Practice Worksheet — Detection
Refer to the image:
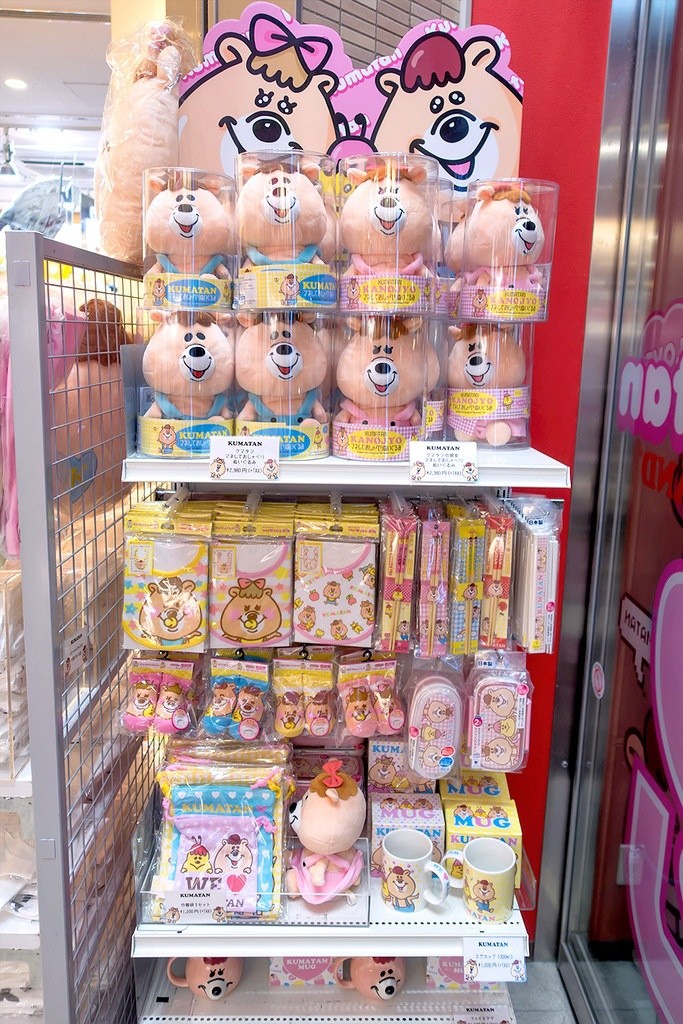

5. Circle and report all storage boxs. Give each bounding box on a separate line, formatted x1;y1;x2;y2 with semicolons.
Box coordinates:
371;792;445;879
367;736;435;797
439;768;510;806
443;799;522;889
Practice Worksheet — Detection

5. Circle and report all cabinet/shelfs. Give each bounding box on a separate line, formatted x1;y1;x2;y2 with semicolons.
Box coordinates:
1;230;168;1024
121;445;570;1024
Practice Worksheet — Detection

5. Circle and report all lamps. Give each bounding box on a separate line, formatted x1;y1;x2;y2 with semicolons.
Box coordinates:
0;127;15;174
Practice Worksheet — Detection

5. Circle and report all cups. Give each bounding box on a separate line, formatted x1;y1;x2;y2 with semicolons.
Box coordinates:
166;958;244;1002
441;837;517;924
382;828;450;911
332;957;406;1001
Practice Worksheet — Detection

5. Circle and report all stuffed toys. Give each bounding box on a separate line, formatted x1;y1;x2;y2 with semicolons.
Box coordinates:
44;299;143;519
231;308;328;428
335;312;425;427
94;43;180;263
467;185;547;289
340;161;426;275
283;757;367;908
144;170;236;278
142;308;232;420
238;159;328;268
445;320;526;448
